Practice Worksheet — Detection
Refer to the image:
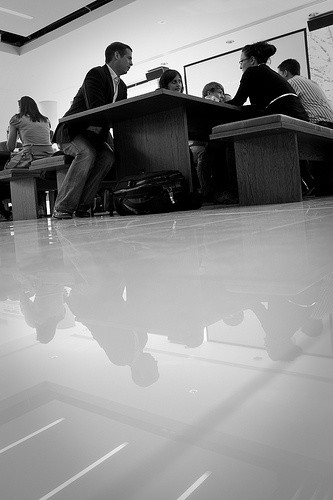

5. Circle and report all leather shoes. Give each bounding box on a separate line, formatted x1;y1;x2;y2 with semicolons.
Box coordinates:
52;207;72;219
75;209;90;217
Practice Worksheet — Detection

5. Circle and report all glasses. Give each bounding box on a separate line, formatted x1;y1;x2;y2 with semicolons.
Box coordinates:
239;57;251;64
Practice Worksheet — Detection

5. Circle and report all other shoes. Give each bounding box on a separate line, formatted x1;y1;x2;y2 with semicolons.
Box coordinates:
38;208;45;217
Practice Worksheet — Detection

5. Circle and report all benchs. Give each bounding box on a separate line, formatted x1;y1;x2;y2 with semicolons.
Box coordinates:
29;155;94;217
0;169;42;221
209;114;333;207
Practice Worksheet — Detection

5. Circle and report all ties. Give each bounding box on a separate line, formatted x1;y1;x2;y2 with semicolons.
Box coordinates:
112;77;119;103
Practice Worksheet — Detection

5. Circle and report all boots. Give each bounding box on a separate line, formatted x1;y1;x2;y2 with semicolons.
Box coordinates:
301;177;315;196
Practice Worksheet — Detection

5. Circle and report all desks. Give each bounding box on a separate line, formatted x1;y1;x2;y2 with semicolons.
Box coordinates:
59;88;263;191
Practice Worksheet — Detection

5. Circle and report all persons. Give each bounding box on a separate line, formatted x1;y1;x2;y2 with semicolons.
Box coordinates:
225;42;317;196
4;96;53;217
277;59;333;129
156;69;202;194
189;82;237;204
51;42;133;218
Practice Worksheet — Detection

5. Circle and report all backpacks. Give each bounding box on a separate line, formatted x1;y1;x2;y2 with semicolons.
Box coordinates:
113;170;202;216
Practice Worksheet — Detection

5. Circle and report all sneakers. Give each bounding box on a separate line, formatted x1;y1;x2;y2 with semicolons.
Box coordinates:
215;190;238;204
